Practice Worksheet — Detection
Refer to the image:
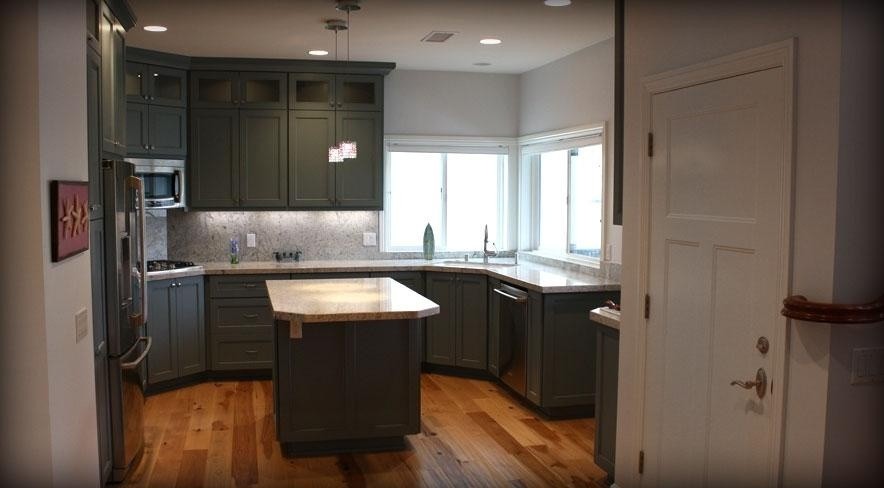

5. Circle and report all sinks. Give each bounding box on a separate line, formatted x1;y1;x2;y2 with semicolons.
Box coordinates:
433;261;521;267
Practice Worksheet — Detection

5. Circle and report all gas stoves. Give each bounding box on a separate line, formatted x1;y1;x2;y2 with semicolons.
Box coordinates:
135;259;199;273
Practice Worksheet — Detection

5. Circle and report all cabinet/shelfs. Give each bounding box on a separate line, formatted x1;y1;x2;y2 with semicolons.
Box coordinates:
87;0;116;487
127;64;187;155
206;275;291;373
142;276;205;393
424;272;488;370
289;72;382;208
98;0;137;160
191;72;287;208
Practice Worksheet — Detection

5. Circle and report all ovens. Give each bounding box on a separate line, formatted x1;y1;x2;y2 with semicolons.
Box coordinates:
493;281;528;399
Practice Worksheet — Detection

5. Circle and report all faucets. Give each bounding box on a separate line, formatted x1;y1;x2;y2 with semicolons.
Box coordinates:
484;224;488;263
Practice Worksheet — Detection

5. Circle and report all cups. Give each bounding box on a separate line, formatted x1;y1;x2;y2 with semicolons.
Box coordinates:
230;253;239;264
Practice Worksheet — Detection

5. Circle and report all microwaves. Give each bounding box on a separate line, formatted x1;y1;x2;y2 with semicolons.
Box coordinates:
125;159;189;209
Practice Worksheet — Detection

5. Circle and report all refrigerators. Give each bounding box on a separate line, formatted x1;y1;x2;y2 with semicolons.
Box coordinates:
103;158;153;472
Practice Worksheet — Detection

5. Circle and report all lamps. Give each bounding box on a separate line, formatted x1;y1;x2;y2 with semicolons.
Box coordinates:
324;0;361;163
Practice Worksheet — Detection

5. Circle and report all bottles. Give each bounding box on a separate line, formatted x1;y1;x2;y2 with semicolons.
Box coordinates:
423;224;435;260
